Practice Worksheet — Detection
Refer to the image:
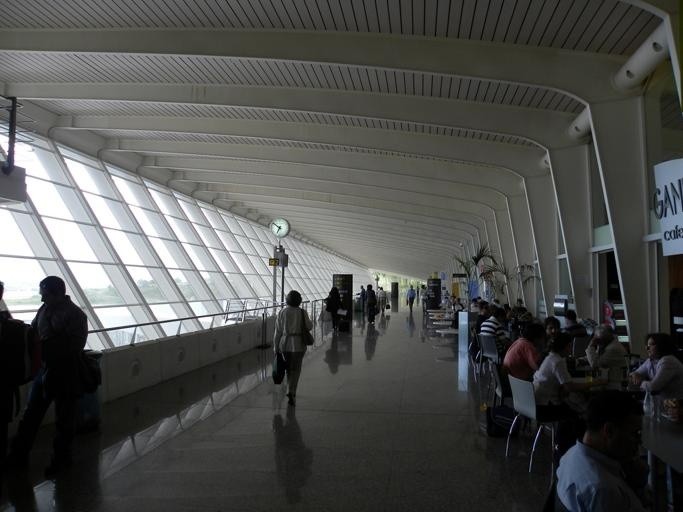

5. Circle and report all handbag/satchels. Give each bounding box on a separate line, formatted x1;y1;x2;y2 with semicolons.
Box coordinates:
386;303;390;309
272;355;285;384
80;357;99;397
302;327;314;346
336;309;348;316
486;405;518;438
367;296;376;304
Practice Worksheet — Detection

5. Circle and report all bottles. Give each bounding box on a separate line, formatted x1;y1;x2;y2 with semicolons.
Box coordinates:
642;387;655;417
619;367;629;388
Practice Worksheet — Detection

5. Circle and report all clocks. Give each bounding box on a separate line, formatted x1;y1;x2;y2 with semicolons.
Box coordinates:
270;217;290;239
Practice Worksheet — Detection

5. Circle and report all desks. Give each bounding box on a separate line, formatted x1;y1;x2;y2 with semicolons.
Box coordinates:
639;415;683;491
427;309;458;337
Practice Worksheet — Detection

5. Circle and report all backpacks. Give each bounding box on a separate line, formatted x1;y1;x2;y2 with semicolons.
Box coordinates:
2;313;43;386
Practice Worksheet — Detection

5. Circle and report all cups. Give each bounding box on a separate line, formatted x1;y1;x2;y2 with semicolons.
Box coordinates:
584;366;593;384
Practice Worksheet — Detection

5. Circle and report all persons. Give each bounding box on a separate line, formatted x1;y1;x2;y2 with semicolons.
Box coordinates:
356;284;389;324
326;287;343;332
0;282;20;512
439;295;683;512
273;290;313;405
419;285;428;312
407;284;416;312
8;276;88;478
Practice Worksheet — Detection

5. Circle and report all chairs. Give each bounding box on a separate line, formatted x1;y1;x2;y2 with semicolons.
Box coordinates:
470;324;593;485
